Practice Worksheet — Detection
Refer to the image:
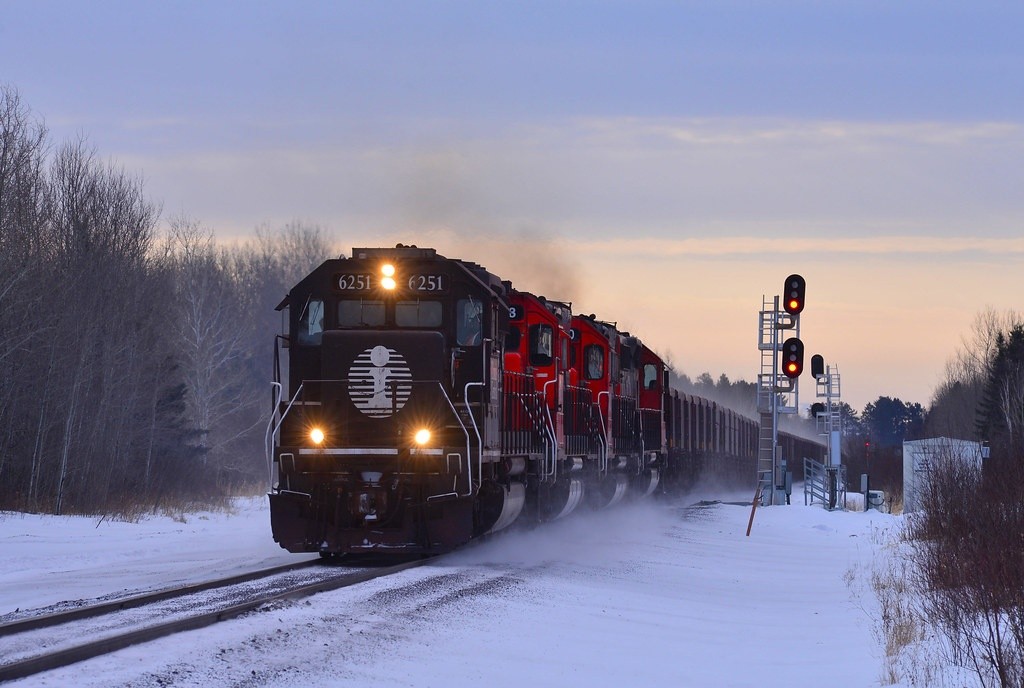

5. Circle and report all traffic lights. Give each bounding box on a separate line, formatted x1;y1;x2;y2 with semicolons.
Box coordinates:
784;275;804;315
864;440;872;458
782;336;803;378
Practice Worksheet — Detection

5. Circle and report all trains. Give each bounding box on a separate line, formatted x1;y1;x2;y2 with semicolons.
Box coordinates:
269;245;826;566
